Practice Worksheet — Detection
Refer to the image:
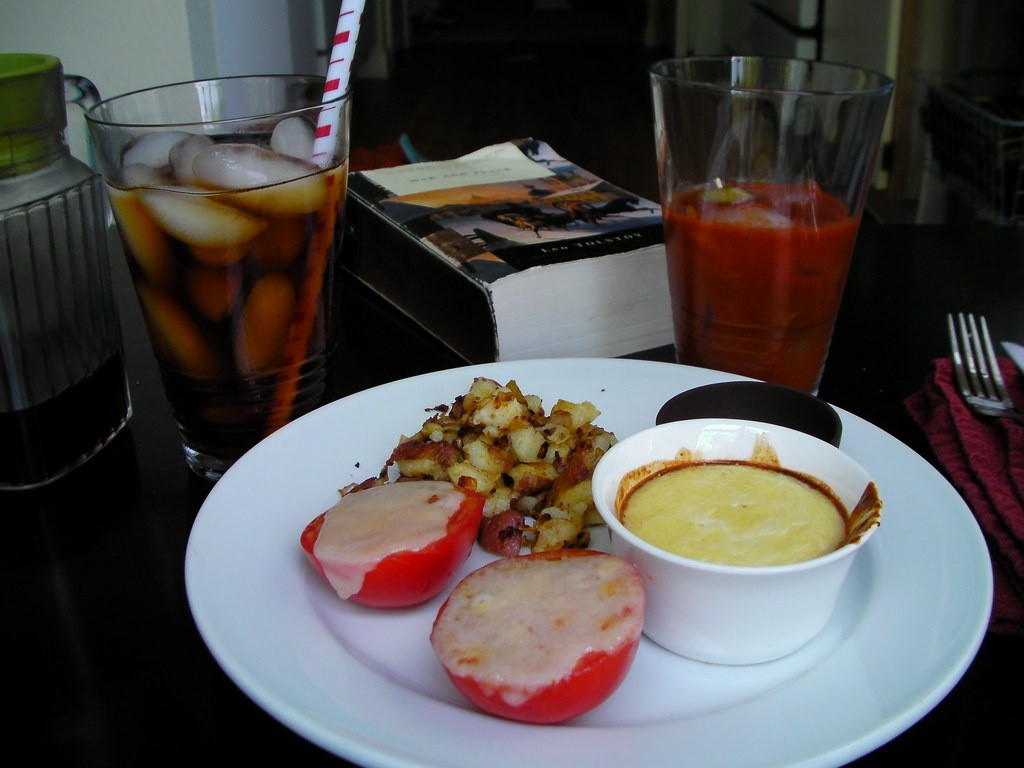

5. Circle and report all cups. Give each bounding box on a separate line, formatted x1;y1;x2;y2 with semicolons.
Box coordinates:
86;73;355;483
648;54;897;400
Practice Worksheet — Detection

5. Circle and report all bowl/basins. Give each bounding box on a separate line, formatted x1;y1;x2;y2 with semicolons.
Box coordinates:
588;418;882;666
656;381;843;454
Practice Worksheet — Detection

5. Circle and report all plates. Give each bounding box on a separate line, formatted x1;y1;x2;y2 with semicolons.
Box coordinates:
182;355;994;768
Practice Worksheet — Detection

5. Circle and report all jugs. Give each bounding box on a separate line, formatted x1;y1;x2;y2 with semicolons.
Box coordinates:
0;53;133;491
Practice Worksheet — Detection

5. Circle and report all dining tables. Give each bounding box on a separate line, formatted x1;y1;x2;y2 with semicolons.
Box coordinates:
0;224;1024;768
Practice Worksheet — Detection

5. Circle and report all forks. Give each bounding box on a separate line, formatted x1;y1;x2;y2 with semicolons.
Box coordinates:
947;311;1024;504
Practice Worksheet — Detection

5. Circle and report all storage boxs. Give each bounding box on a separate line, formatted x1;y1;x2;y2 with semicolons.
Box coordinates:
907;70;1023;227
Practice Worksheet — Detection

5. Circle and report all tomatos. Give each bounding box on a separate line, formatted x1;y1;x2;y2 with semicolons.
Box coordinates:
429;548;648;723
299;479;485;608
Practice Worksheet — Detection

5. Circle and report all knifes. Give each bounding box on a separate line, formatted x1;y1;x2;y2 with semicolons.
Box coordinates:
999;340;1024;374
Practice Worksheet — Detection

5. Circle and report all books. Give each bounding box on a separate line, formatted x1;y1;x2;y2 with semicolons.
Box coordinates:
339;136;688;370
